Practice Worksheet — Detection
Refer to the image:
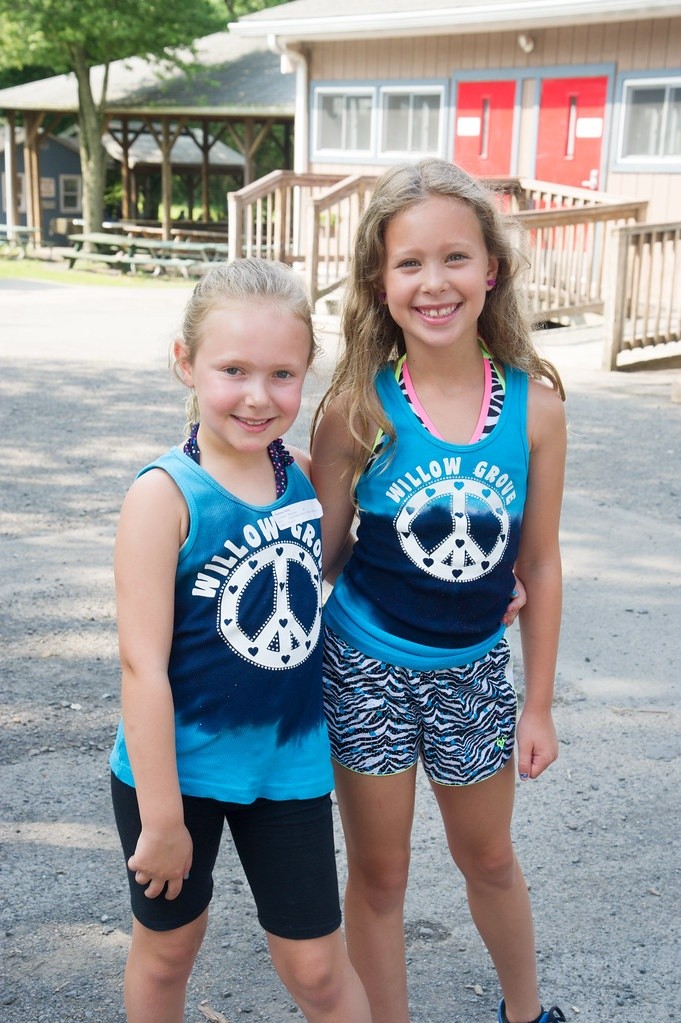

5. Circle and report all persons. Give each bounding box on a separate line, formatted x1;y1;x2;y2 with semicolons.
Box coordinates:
311;157;573;1023
104;257;527;1023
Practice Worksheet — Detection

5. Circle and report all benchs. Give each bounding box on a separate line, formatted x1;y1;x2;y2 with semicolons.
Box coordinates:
0;216;293;279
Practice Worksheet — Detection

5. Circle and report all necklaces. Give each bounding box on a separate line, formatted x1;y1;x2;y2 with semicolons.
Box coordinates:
184;422;294;502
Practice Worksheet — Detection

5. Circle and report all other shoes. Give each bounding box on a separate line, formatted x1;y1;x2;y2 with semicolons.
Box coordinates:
496;997;567;1023
